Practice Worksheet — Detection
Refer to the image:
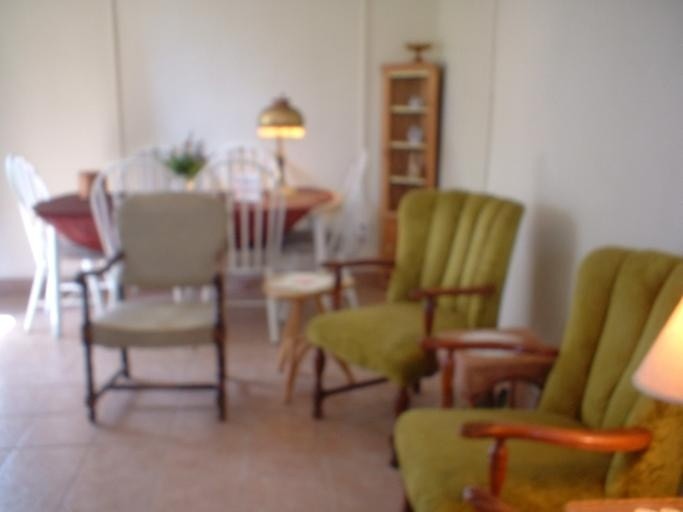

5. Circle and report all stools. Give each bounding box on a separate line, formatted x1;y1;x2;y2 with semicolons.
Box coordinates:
261;271;355;402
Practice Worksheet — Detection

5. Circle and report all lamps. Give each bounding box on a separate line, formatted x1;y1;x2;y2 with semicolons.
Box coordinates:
254;96;305;200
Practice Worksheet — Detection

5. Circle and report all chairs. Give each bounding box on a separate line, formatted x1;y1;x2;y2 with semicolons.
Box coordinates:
304;189;523;470
74;192;230;422
388;243;683;511
4;138;366;343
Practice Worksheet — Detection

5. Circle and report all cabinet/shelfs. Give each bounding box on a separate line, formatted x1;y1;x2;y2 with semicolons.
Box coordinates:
379;62;443;276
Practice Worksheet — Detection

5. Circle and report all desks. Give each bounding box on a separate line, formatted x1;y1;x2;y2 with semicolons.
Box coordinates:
434;321;559;415
